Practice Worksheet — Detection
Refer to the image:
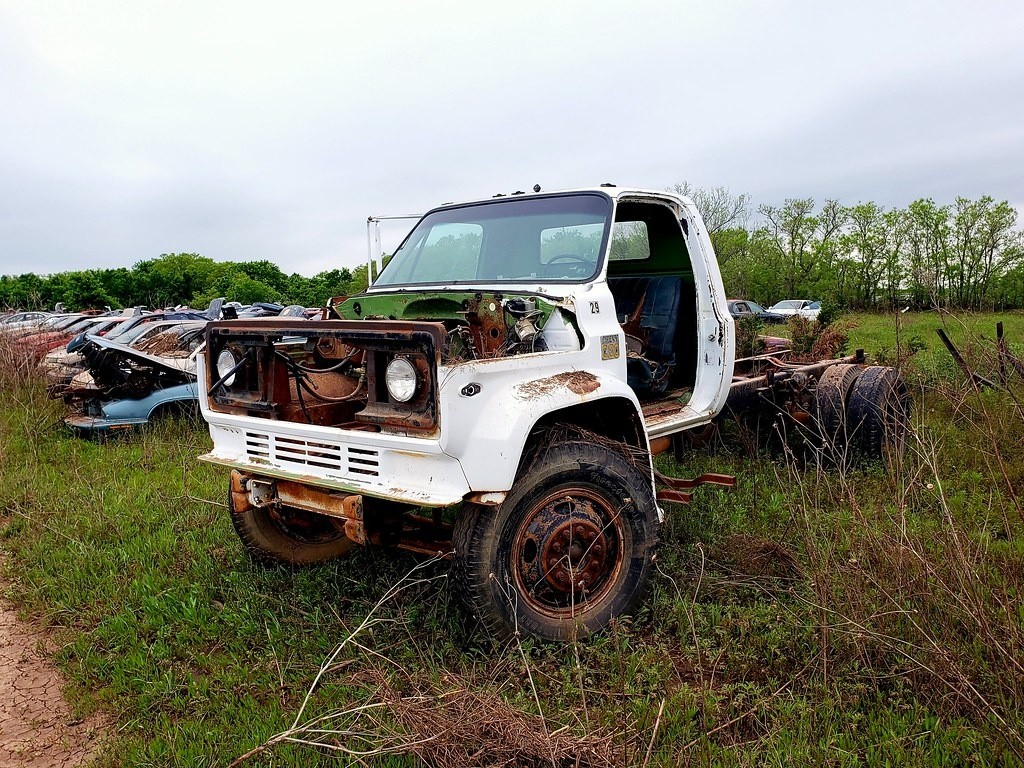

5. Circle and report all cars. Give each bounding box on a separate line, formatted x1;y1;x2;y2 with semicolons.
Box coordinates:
726;299;790;326
764;299;823;322
0;296;337;446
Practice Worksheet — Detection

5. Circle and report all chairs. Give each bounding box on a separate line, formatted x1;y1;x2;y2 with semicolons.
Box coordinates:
618;274;683;400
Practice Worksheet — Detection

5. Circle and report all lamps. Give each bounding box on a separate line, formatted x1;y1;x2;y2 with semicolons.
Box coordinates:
383;356;424;404
216;348;246;388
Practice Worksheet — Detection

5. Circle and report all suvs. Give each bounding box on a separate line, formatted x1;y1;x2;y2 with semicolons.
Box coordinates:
186;183;910;661
